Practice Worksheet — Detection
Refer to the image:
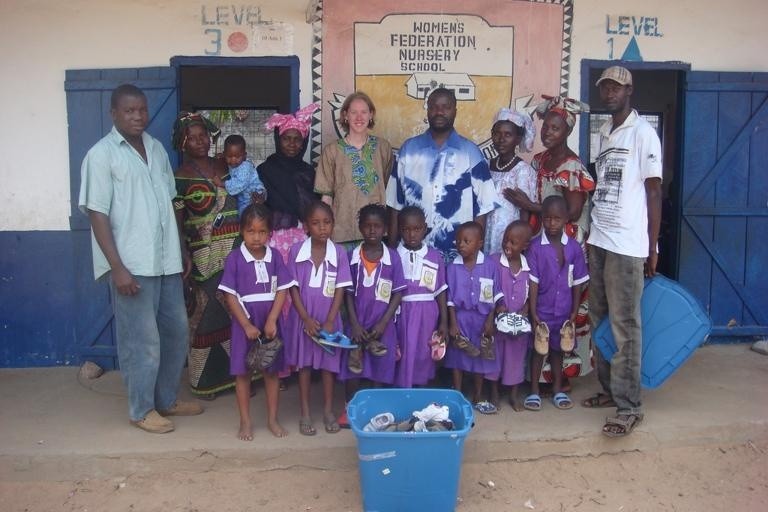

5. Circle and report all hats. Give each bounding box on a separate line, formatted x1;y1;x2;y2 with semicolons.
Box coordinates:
595;66;633;89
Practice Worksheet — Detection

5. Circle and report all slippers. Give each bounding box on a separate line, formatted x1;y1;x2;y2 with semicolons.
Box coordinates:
299;420;316;435
324;419;340;433
523;394;542;411
549;393;573;409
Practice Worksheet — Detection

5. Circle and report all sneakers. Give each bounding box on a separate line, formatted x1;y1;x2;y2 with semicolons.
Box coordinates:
495;312;532;336
413;403;448;423
480;333;496;360
129;408;174;434
363;413;395;432
156;399;204;416
451;334;480;358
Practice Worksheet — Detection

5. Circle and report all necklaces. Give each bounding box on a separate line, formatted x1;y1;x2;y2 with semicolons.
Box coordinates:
346;136;367;151
496;154;517;171
189;160;212;178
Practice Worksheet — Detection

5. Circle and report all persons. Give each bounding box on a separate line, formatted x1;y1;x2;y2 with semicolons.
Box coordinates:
337;204;408;428
482;108;537;251
173;112;269;401
218;203;292;441
582;66;663;437
76;84;205;435
313;91;395;252
524;195;590;410
501;96;596;399
446;221;504;417
214;134;268;218
491;220;530;413
395;206;450;388
256;102;317;392
384;89;501;264
286;199;354;435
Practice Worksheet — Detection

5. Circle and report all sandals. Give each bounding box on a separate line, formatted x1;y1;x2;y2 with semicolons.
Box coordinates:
428;330;447;362
582;393;617;408
302;328;358;356
364;336;389;357
246;334;283;372
347;341;364;375
395;344;402;362
602;413;644;438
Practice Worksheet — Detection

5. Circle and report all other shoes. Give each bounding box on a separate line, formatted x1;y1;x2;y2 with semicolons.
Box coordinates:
387;418;454;433
473;401;497;414
337;413;351;429
559;320;576;352
534;322;550;355
